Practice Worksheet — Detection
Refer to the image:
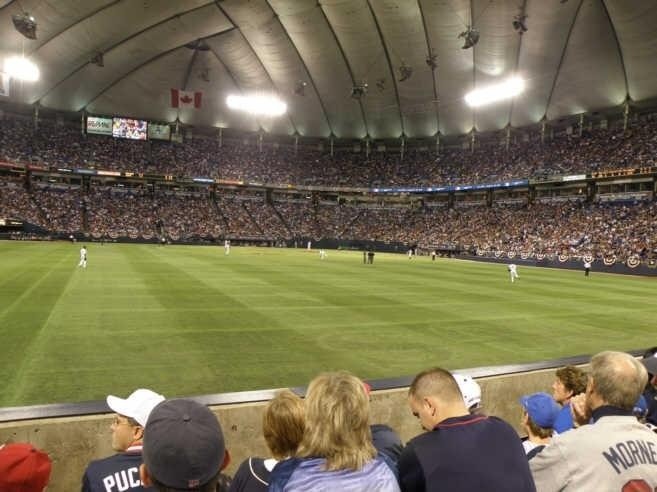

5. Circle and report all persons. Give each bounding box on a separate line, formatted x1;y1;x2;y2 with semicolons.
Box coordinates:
1;112;657;264
78;245;87;268
507;264;520;285
584;261;592;278
0;349;657;492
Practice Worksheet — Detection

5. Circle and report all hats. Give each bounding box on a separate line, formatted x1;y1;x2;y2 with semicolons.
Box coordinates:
520;392;560;427
0;443;52;492
106;389;166;429
453;374;481;410
141;400;226;486
632;392;647;413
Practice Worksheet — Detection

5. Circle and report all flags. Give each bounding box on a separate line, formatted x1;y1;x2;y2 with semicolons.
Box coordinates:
169;87;203;109
